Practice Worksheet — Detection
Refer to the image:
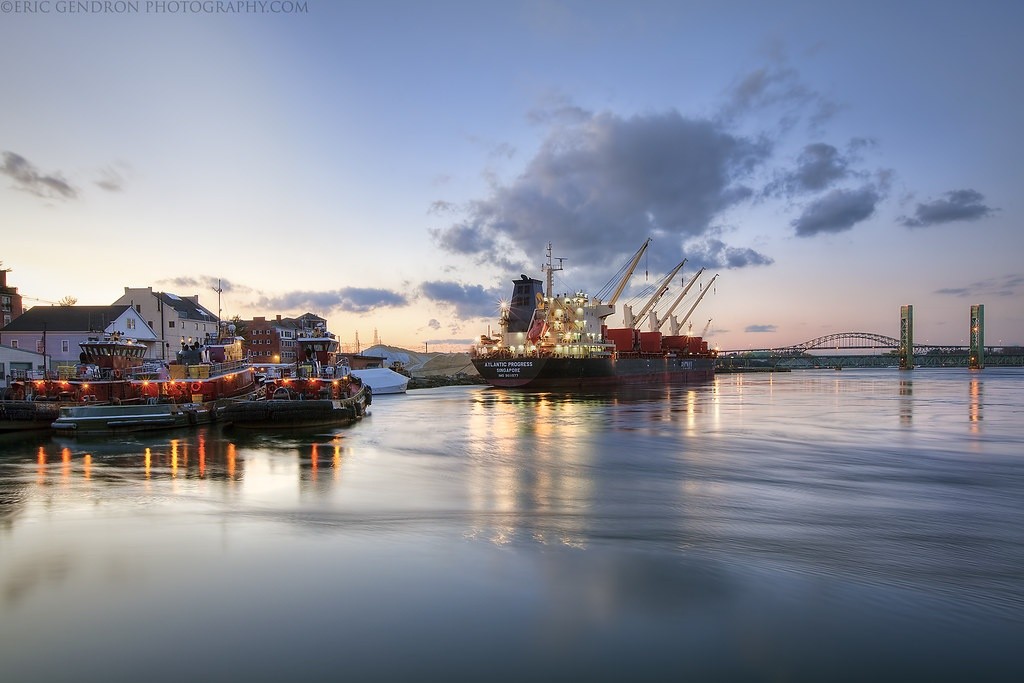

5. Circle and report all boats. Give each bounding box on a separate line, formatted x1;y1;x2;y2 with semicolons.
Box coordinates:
50;276;267;434
466;241;718;390
212;371;373;430
0;320;169;432
527;319;550;346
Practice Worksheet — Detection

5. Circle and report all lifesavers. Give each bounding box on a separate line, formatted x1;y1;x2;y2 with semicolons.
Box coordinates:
190;382;202;393
115;369;120;378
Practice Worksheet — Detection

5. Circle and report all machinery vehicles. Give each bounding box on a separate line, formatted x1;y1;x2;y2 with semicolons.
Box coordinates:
620;257;688;355
582;236;655;352
645;267;719;356
